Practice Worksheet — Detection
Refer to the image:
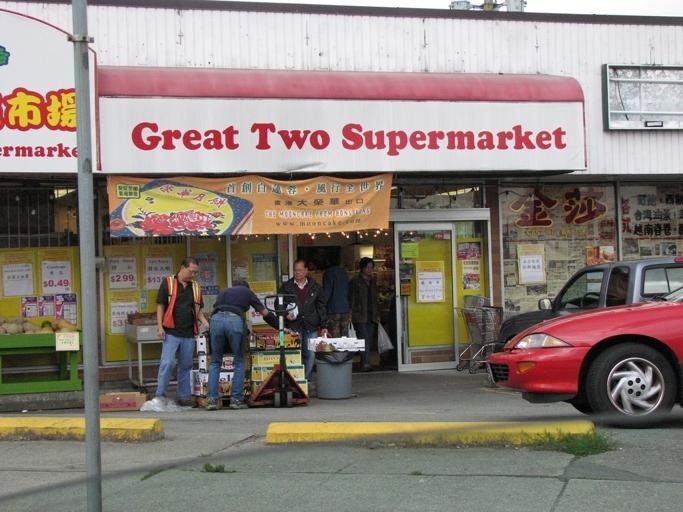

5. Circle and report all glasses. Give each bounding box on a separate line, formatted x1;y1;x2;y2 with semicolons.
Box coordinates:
186;268;199;276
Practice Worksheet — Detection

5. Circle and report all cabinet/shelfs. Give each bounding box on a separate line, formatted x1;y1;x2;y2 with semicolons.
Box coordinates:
0;329;83;394
125;324;178;388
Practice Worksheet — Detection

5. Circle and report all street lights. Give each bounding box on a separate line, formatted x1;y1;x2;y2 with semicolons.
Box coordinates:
448;0;527;14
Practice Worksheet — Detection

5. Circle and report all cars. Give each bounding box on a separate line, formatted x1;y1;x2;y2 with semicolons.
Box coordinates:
489;285;683;429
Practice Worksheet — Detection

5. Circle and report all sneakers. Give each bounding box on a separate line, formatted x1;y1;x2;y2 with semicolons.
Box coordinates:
204;396;218;410
176;399;198;408
229;398;249;409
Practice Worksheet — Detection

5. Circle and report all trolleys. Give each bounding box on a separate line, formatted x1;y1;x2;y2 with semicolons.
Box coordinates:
456;304;516;375
247;294;311;408
124;320;178;395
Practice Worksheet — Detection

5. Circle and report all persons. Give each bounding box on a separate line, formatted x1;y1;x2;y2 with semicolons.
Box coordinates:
274;259;327;383
155;257;210;408
206;280;289;410
321;254;351;338
348;257;381;371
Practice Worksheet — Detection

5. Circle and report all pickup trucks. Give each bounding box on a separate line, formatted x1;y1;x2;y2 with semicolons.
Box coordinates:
497;257;683;352
